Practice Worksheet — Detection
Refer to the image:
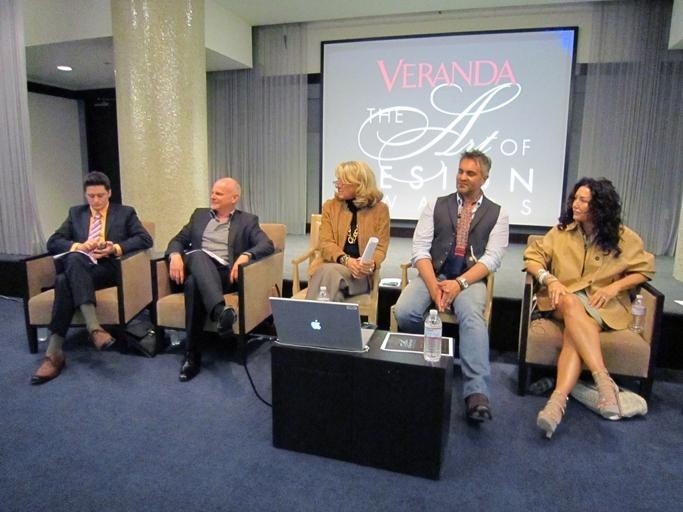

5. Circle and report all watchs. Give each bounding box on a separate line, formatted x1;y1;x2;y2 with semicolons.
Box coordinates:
456;277;469;290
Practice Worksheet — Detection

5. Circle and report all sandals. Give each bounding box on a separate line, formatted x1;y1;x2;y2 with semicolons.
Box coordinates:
592;368;622;421
536;391;570;438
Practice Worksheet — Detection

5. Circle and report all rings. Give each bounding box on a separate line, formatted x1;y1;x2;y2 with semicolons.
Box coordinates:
602;296;606;300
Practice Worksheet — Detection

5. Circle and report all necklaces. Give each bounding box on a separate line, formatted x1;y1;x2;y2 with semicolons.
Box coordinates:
347;222;358;245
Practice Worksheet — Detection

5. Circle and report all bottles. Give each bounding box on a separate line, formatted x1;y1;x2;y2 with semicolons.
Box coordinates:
629;294;647;334
423;310;443;363
316;286;330;302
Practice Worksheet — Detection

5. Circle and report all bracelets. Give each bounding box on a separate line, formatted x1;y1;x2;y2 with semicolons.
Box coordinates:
339;254;350;265
536;269;546;280
539;271;549;286
243;252;253;260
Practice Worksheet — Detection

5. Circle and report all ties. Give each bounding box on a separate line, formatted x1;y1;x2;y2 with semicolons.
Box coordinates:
87;213;102;248
456;201;474;257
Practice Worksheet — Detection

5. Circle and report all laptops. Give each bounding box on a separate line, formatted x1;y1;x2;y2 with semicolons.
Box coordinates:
268;297;374;349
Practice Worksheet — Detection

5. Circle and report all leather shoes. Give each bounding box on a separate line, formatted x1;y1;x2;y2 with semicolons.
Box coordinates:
177;356;202;383
217;308;236;341
466;395;492;422
91;328;116;352
30;355;67;383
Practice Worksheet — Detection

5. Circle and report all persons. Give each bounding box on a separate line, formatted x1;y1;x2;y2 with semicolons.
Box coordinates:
303;160;390;303
30;172;152;385
392;149;510;422
162;178;274;381
520;176;655;439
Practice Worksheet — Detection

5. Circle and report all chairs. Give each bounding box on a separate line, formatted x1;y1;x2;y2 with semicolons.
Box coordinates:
514;230;667;396
290;212;383;329
19;219;159;356
149;221;286;366
386;255;494;360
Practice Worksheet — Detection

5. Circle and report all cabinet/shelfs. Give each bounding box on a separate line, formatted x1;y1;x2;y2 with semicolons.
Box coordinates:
268;330;456;480
